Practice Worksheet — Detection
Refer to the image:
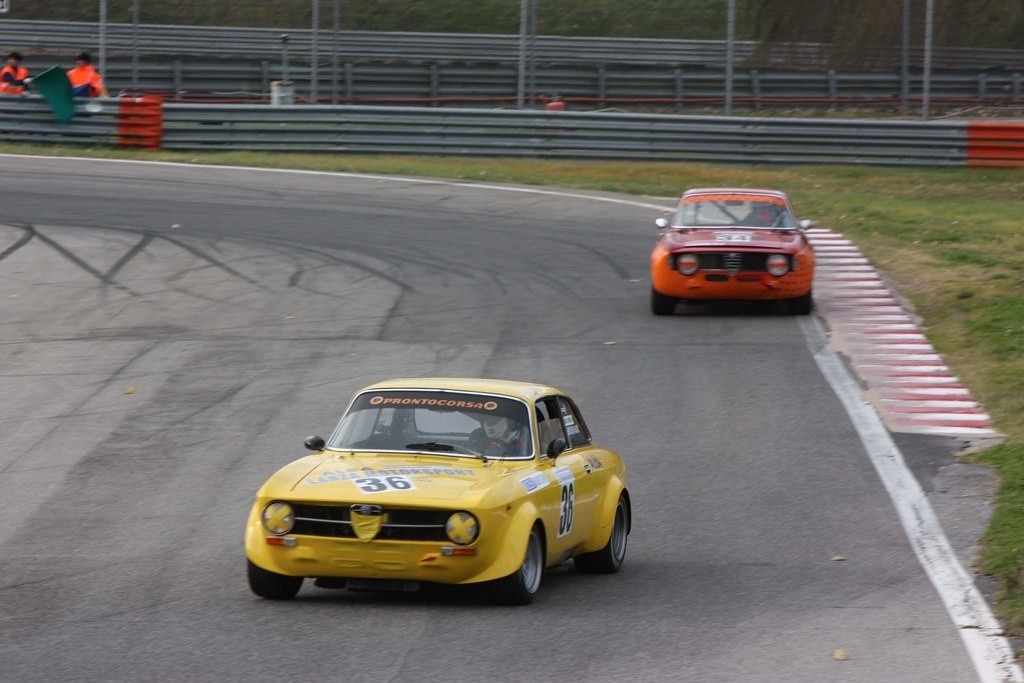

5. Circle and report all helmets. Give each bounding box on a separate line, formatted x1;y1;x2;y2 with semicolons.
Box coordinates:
752;199;779;226
483;398;520;446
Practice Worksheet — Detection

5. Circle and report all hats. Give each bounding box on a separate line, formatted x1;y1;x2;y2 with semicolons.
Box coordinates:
75;51;89;61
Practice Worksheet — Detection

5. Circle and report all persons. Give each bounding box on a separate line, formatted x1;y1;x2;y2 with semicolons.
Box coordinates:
66;53;109;97
0;51;30;96
470;415;523;455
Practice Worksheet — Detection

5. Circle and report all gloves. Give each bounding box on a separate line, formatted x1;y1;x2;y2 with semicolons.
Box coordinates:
23;91;30;95
23;77;33;84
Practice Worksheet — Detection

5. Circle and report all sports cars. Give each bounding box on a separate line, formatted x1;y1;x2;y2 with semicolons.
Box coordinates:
244;376;632;606
649;186;818;314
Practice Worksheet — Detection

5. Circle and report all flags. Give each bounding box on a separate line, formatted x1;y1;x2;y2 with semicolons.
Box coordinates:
33;66;75;123
